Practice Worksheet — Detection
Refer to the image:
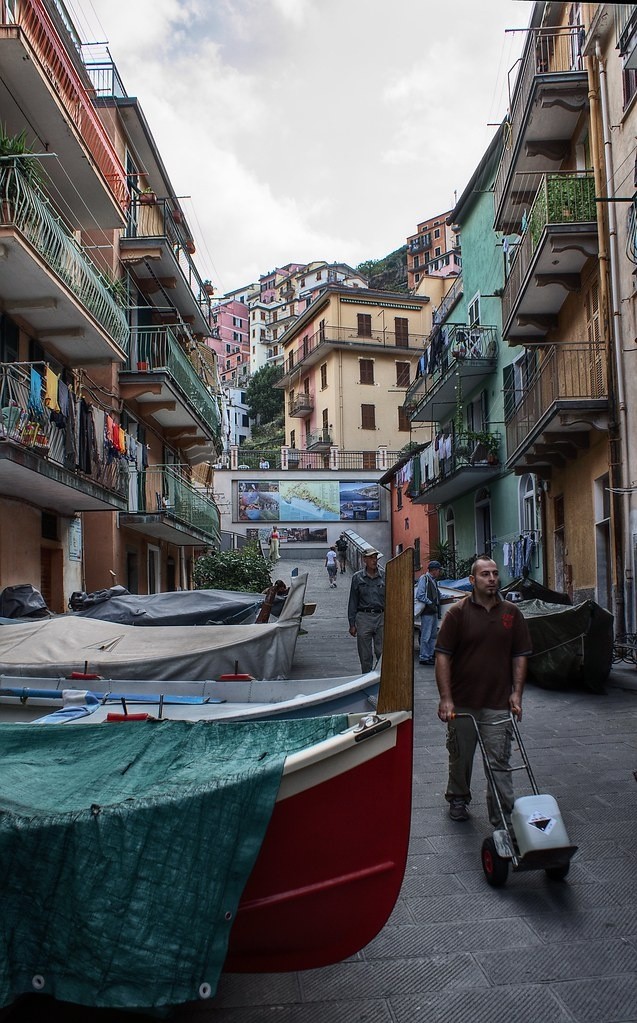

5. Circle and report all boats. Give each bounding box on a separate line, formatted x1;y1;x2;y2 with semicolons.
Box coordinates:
0;548;418;977
0;572;308;682
494;569;614;693
0;652;382;725
70;568;298;626
411;581;472;632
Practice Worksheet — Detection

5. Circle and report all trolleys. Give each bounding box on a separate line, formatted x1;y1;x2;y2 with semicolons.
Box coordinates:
451;710;579;888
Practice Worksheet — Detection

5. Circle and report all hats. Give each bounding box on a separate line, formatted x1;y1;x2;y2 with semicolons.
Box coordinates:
427;561;446;571
362;548;380;556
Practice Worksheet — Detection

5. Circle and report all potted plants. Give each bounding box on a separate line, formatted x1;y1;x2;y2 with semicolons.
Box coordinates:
487;446;499;463
136;352;149;369
139;187;157;203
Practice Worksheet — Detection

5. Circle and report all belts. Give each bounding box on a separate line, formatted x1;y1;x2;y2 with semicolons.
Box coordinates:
359;608;384;614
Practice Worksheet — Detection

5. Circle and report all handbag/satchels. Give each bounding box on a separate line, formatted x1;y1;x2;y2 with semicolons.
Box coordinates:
414;574;429;619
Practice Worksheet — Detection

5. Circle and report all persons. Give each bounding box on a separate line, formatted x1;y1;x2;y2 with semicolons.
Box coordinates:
268;525;284;566
334;534;349;574
416;561;455;664
347;548;386;674
259;457;270;469
434;555;533;844
325;547;338;588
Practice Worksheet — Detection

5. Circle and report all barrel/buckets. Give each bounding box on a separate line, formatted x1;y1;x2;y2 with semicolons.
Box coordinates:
137;362;148;370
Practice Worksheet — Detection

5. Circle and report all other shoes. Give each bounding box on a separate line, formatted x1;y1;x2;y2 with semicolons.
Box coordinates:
420;659;435;665
448;800;469;821
330;585;333;588
333;581;337;587
